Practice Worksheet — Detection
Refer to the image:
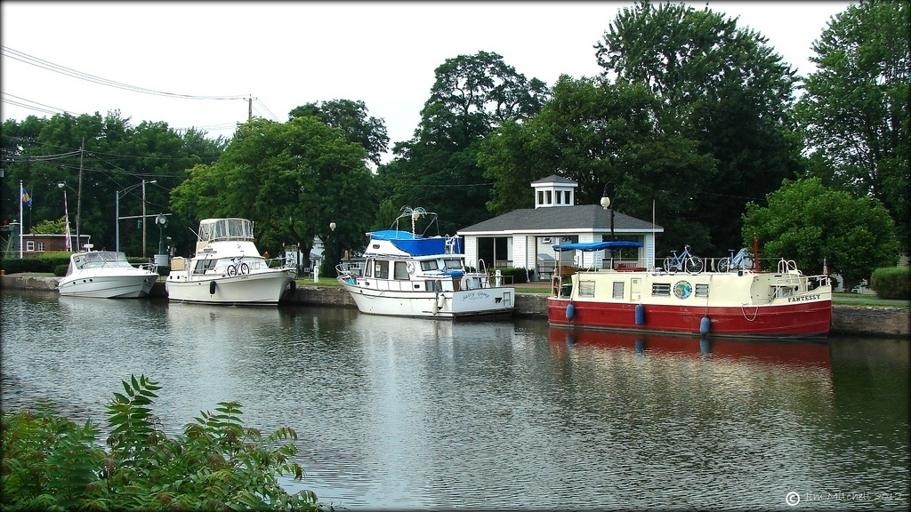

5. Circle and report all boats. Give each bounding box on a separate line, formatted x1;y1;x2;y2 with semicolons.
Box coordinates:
165;217;297;307
545;241;832;339
55;238;160;299
335;205;515;319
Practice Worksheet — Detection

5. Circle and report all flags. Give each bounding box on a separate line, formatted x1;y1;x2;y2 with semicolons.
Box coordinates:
21;189;35;208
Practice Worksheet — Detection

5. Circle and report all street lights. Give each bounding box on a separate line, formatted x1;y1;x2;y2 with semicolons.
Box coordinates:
599;178;616;264
116;179;158;252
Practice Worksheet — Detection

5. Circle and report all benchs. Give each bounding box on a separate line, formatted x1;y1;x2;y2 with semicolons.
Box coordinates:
556;265;576;297
